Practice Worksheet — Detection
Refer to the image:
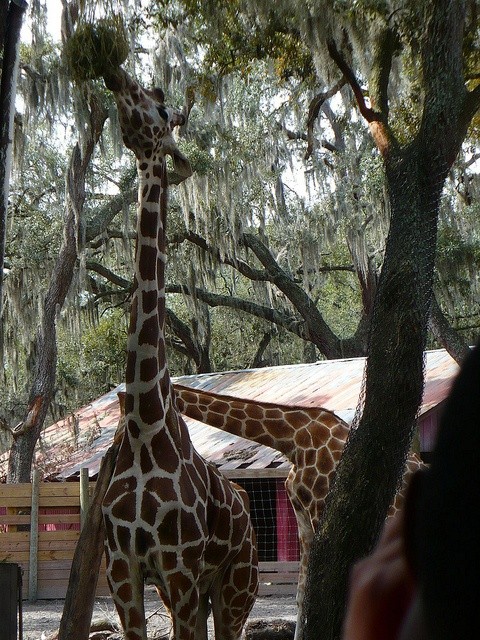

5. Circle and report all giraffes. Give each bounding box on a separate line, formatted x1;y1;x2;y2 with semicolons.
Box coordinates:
114;378;429;640
99;63;261;639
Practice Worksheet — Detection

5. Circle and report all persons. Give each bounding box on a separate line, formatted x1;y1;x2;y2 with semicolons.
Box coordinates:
342;340;480;639
